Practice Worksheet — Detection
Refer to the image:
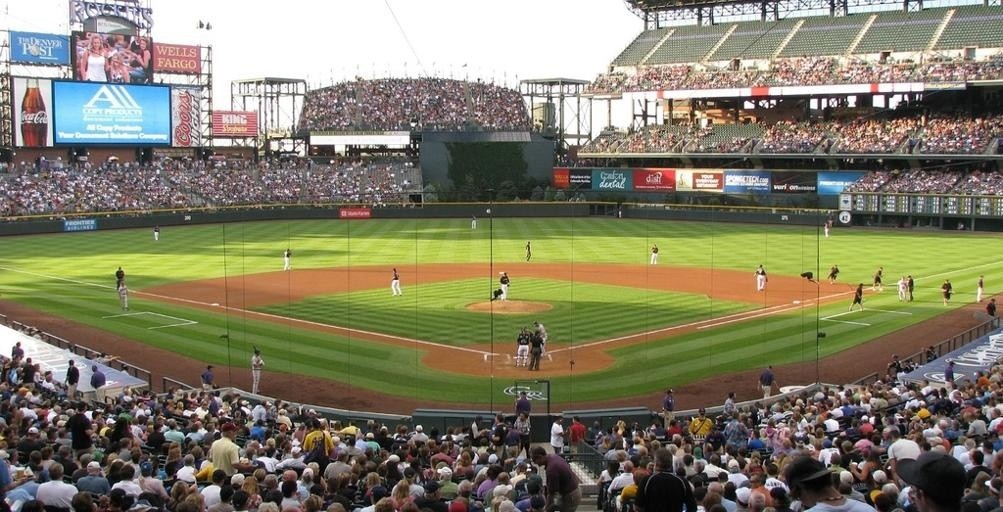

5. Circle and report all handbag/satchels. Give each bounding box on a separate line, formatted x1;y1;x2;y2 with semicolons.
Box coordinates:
303;441;326;464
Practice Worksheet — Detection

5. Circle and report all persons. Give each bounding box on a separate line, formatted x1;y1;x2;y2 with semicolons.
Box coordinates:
490;284;511;301
872;266;954;309
753;264;768;292
650;244;659;264
115;265;125;292
0;319;1002;511
471;212;478;230
985;296;997;320
282;247;293;272
976;274;985;302
849;282;865;313
1;75;534;219
580;50;1003;237
391;267;404;297
499;270;511;301
118;281;130;310
153;224;161;241
525;240;533;262
77;33;153;85
798;266;841;286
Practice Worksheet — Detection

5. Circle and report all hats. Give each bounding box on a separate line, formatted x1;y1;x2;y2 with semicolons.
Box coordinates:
19;387;30;391
896;451;965;499
28;427;39;434
437;467;452;475
80;451;104;469
221;422;240;433
823;440;832;448
699;408;705;414
735;487;751;503
840;468;898;511
106;418;116;424
785;457;832;485
861;415;868;421
694;447;702;458
366;433;374;438
389;454;400;463
728;459;739;468
488;453;527;495
424;481;444;492
416;425;423;431
